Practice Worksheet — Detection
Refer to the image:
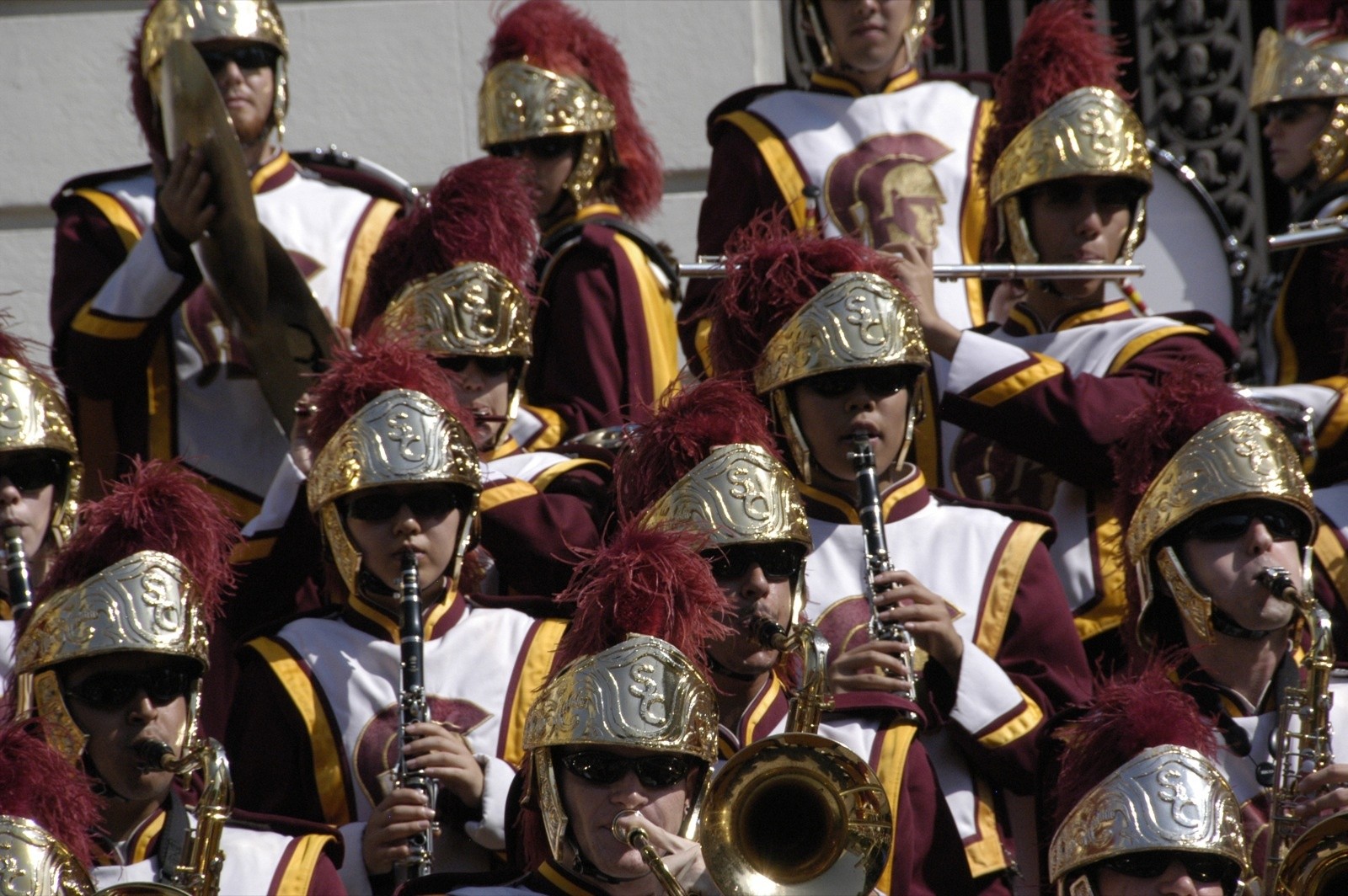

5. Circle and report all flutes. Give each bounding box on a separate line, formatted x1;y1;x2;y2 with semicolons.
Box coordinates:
1264;216;1348;253
675;253;1143;279
4;528;40;732
396;550;432;875
845;428;921;721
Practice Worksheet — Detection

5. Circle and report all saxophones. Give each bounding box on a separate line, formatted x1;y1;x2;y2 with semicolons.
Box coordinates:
95;739;231;896
1256;565;1348;896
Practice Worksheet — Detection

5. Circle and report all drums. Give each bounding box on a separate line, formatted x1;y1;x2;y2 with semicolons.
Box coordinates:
1009;132;1247;337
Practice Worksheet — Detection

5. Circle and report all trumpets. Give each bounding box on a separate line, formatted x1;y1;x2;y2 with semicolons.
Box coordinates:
612;737;894;895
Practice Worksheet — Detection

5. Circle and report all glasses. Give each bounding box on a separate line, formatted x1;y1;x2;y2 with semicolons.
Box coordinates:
1089;853;1237;886
1267;100;1334;128
67;664;193;708
430;355;519;375
490;132;581;166
558;746;701;792
794;367;914;401
1171;504;1305;544
1026;182;1137;213
343;483;468;524
696;543;799;588
197;45;277;77
0;457;68;490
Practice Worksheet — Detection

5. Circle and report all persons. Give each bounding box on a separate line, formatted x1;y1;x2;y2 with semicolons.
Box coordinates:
0;0;1348;896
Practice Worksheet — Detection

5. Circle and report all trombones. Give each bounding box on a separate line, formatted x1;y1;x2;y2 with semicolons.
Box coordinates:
700;615;894;735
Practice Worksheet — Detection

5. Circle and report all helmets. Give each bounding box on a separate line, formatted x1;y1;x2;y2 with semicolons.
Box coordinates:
132;0;289;142
0;290;83;549
1248;0;1348;184
977;0;1155;284
517;499;740;862
477;0;664;219
367;153;552;424
616;346;813;633
677;193;931;482
1114;363;1319;641
0;715;97;896
789;0;935;73
3;448;250;771
305;336;483;598
1048;643;1249;896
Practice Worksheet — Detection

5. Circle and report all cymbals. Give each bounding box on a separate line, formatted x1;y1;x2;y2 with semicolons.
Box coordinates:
164;43;349;442
